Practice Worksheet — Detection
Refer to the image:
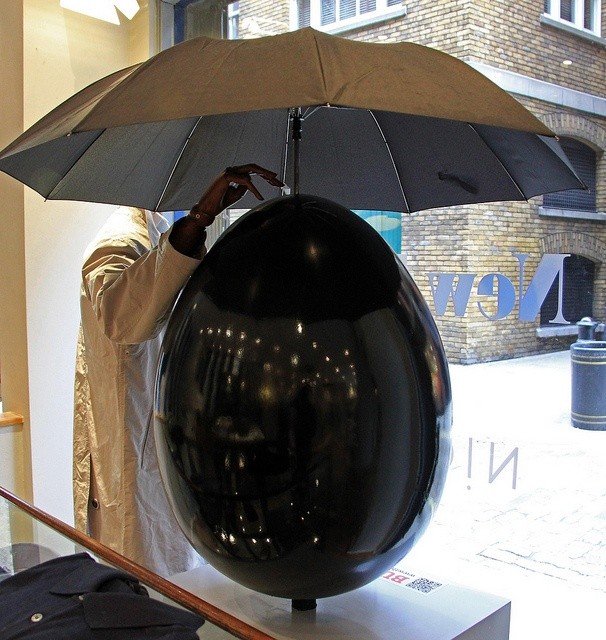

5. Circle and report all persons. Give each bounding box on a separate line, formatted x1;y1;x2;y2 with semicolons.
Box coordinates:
72;162;285;587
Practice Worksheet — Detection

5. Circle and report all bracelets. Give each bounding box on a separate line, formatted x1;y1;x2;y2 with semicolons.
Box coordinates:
190;205;215;227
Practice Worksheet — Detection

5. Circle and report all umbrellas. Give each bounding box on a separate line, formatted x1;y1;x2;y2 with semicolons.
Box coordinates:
0;27;588;215
